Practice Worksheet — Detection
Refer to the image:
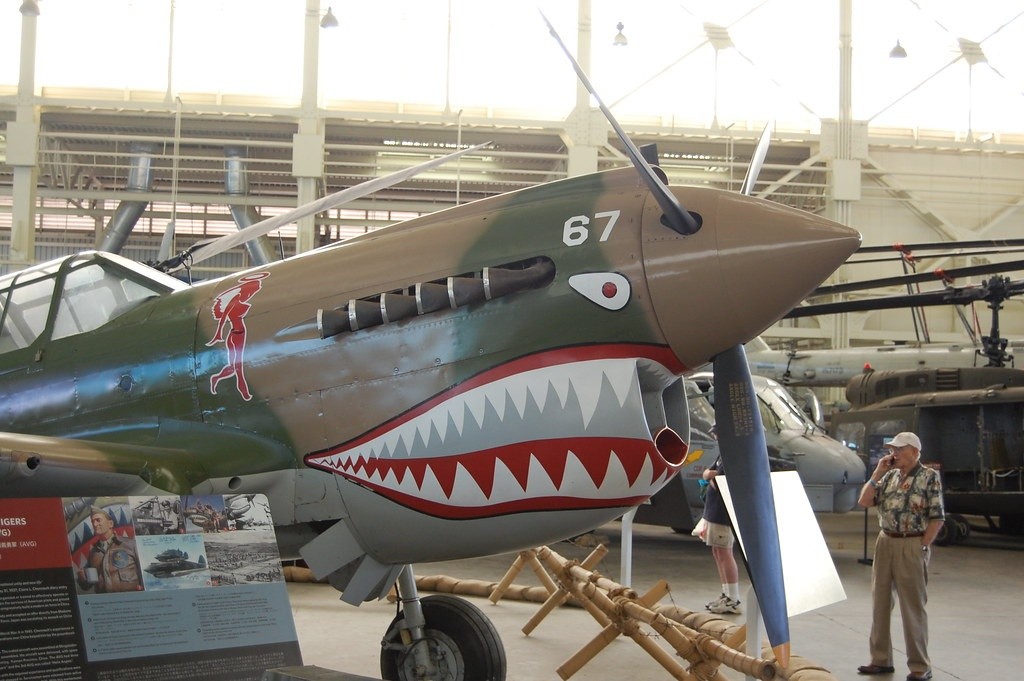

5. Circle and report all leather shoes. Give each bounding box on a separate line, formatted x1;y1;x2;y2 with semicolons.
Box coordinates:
906;670;932;681
857;664;895;673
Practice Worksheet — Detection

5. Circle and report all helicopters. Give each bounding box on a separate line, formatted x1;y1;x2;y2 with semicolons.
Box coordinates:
844;366;1023;404
829;387;1024;547
0;166;864;681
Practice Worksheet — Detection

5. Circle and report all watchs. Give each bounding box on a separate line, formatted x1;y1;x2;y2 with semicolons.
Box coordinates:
921;545;928;552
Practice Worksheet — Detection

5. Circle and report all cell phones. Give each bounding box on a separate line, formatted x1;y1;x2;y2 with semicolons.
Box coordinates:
890;458;895;467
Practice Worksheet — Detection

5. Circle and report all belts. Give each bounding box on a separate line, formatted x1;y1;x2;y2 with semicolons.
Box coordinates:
882;528;925;538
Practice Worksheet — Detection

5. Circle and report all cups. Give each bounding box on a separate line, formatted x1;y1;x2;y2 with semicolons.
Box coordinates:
83;568;99;584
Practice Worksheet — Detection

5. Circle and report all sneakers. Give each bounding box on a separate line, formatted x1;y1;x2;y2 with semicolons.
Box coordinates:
704;592;743;614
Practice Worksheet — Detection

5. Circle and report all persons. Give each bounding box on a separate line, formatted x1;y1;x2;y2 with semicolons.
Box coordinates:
76;504;144;593
857;432;945;681
703;421;742;614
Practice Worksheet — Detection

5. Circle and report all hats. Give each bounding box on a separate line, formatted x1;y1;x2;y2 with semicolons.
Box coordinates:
883;432;921;451
707;424;718;434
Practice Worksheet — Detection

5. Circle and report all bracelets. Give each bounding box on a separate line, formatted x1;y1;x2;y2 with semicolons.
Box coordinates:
869;478;877;489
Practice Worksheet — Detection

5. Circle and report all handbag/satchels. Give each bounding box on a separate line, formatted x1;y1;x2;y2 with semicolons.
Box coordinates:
697;478;710;503
692;518;708;543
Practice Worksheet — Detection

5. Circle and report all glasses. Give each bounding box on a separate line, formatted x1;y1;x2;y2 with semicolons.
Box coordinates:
889;447;904;453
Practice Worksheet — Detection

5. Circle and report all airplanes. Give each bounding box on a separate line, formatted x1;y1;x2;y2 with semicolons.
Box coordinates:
697;336;1013;385
668;374;867;529
